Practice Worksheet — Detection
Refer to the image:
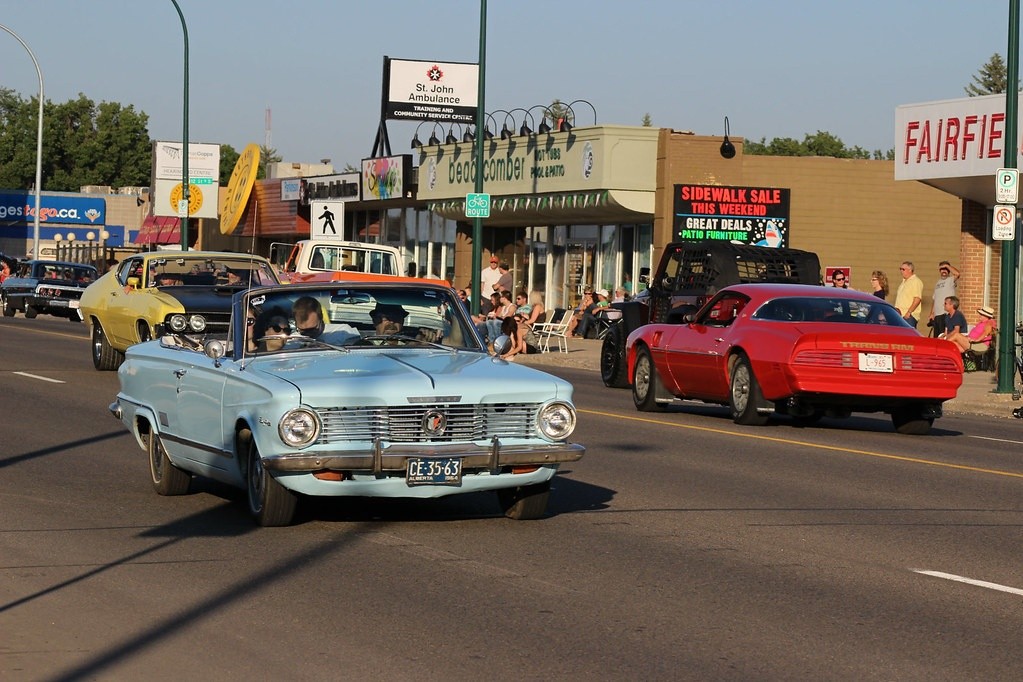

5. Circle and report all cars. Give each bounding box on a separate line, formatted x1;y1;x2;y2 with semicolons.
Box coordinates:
108;281;586;526
624;283;964;438
76;249;330;371
2;259;100;323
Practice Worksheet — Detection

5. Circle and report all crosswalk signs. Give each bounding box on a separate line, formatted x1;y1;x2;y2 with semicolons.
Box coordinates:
311;199;344;242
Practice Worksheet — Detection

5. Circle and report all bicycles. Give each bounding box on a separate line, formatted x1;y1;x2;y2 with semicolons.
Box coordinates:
993;324;1023;386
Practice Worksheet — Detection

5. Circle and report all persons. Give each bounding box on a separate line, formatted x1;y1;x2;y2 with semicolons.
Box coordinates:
0;261;10;306
865;270;889;324
246;296;359;354
227;269;244;286
831;270;857;314
894;261;923;329
353;288;466;348
562;272;632;340
457;256;547;361
929;261;994;354
123;263;184;295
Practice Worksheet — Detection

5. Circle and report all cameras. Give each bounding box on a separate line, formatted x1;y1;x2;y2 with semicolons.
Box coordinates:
927;318;937;327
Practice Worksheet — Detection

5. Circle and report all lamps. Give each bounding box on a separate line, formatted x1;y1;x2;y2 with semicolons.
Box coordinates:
463;112;497;143
560;100;596;132
429;121;462;146
720;117;736;159
135;197;146;207
446;122;471;145
501;108;534;140
410;121;445;148
483;110;515;140
520;105;554;137
539;102;575;135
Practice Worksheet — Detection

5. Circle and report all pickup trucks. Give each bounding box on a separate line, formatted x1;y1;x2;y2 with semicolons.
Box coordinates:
269;240;451;343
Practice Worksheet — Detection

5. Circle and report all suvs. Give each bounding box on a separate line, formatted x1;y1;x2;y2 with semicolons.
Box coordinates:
599;240;822;389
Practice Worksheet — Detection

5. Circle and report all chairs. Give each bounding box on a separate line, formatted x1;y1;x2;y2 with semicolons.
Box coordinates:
530;309;574;353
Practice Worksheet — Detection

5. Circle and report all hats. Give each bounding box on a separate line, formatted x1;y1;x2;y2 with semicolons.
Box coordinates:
369;302;409;318
977;307;995;318
596;289;609;297
489;256;498;262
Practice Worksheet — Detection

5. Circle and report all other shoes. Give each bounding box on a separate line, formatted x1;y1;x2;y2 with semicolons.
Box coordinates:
570;335;584;339
487;343;514;362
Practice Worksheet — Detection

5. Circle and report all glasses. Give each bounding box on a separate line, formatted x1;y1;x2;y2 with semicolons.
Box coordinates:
490;262;496;263
835;277;845;281
584;293;592;295
615;290;619;293
515;320;520;322
945;302;946;305
296;320;321;335
247;318;257;326
872;278;879;281
516;298;521;300
900;267;905;271
378;313;404;322
272;325;294;335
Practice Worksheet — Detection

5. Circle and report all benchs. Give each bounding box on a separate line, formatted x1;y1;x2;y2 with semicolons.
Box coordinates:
928;325;996;372
